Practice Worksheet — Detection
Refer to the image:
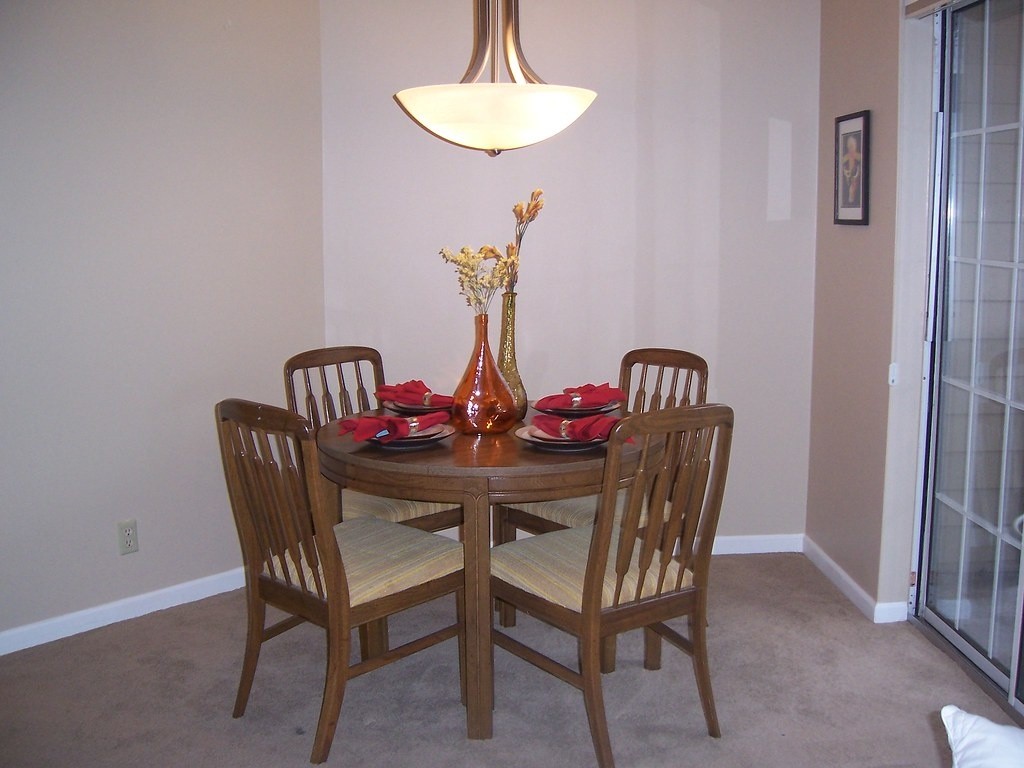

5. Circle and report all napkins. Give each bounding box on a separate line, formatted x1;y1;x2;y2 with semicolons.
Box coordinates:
536;382;627;411
338;411;451;444
532;415;635;444
374;380;454;406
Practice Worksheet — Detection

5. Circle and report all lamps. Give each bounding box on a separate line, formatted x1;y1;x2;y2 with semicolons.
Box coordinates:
394;0;598;158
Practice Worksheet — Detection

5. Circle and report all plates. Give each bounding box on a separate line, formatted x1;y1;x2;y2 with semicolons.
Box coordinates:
530;399;623;419
363;423;456;452
514;425;609;453
382;400;453;416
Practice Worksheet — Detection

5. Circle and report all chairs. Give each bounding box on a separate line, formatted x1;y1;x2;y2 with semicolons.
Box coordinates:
500;348;709;675
489;403;734;768
214;398;468;766
285;345;464;653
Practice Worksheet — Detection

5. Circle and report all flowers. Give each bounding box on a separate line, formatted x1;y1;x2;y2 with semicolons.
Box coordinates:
440;245;520;315
476;187;542;293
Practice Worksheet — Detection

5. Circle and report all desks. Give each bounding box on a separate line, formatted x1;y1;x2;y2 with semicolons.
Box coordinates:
315;399;669;740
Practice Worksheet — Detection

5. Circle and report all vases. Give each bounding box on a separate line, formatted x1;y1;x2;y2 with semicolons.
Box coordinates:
498;293;527;422
450;312;519;434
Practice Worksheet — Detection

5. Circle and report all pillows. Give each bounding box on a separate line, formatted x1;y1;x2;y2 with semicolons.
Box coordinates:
940;705;1023;768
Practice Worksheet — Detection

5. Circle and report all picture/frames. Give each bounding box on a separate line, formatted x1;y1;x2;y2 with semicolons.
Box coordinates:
833;110;870;227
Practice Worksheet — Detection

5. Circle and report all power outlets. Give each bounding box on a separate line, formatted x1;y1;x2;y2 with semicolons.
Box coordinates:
116;520;141;555
